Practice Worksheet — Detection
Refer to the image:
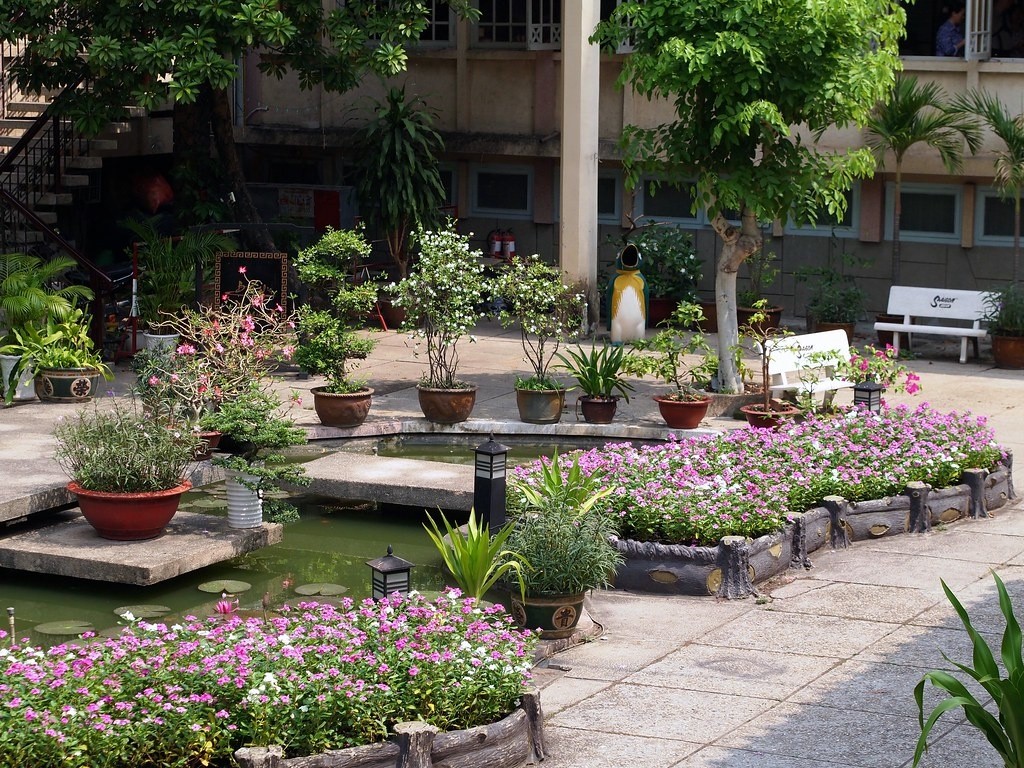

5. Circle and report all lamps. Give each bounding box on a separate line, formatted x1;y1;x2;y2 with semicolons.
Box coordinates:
849;380;882;417
366;543;414;607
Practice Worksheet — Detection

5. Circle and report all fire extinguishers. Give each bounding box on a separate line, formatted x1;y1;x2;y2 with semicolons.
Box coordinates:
487;228;503;259
503;228;516;262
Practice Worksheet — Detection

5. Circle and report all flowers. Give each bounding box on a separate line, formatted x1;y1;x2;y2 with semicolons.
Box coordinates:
0;594;537;762
151;284;288;406
624;223;702;294
394;228;581;346
509;347;1010;550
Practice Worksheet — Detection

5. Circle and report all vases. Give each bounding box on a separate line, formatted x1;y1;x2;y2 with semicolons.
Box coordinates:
648;302;677;324
162;426;220;460
417;381;565;425
698;297;717;332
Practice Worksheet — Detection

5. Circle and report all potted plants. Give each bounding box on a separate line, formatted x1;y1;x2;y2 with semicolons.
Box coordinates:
563;300;808;424
988;297;1024;371
62;402;300;534
1;230;179;401
421;448;626;638
738;241;913;345
294;226;377;425
383;89;427;327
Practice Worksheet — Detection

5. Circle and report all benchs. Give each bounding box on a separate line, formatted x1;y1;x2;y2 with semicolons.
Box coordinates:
772;285;999;405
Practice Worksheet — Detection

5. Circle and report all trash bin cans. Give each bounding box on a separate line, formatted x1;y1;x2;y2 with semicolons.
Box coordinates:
606;244;650;347
